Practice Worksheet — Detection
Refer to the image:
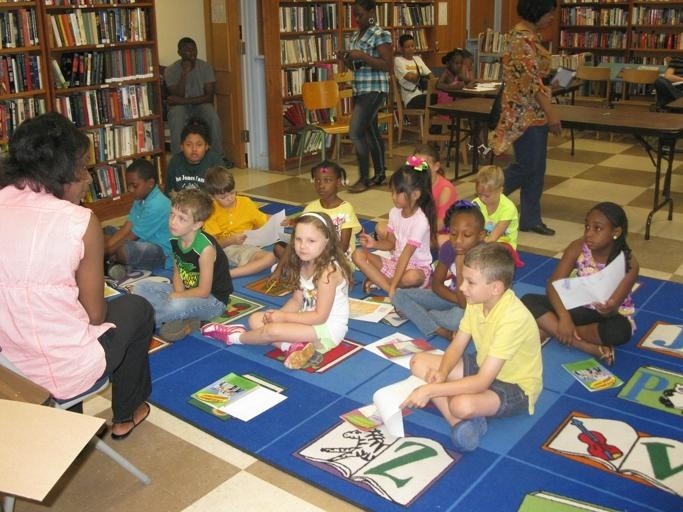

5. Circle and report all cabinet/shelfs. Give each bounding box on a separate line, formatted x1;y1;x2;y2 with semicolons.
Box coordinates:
341;1;392;155
624;2;682;97
392;2;437;145
42;2;165;222
551;2;630;102
259;0;338;174
0;0;52;157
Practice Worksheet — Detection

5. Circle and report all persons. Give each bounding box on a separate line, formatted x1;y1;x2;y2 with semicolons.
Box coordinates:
519;202;640;367
375;145;459;246
335;1;392;192
132;187;233;342
203;166;291;277
395;35;442;149
488;1;562;235
104;159;176;278
271;163;363;273
163;36;231;167
164;124;214;200
655;50;683;105
392;200;486;339
398;241;543;453
472;166;519;249
201;212;354;369
436;51;482;120
352;165;439;294
1;112;155;440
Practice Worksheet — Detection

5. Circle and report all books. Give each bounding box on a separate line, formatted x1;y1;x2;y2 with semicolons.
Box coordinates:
567;0;682;95
0;0;165;203
342;3;389;132
339;399;411;434
378;338;435;357
278;5;335;159
562;358;625;393
393;5;435;56
191;371;287;423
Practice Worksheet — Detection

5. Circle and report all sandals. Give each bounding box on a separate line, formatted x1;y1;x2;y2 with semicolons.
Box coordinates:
362;277;381;294
111;401;150;439
598;344;614;366
466;143;491;158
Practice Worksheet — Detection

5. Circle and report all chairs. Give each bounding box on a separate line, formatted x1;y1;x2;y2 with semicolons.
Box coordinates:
422;72;482;167
390;66;438;145
296;80;352;174
327;72;395;162
569;65;611;139
608;66;659;141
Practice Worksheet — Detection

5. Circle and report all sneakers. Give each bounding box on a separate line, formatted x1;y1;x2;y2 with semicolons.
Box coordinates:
451;416;487;451
106;263;133;280
283;341;315;369
159;317;246;346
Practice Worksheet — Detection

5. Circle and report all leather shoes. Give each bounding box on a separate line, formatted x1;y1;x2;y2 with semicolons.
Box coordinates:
520;222;555;235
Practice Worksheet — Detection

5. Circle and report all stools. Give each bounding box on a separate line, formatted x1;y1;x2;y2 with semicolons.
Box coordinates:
2;374;152;512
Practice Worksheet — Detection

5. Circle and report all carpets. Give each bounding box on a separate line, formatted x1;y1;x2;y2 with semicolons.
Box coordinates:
104;193;682;511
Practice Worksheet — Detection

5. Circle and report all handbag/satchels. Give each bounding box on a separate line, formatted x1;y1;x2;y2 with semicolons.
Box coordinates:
419;77;427;90
487;82;504;131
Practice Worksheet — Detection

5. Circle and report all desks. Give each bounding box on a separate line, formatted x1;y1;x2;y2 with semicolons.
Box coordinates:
665;97;683;112
425;97;682;240
0;360;52;406
444;77;586;167
